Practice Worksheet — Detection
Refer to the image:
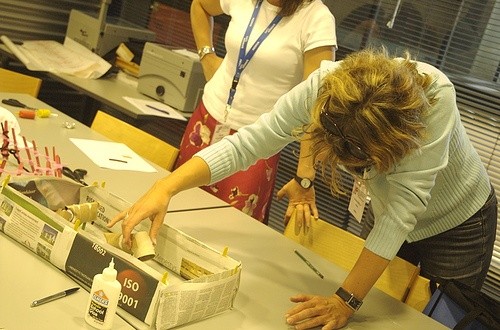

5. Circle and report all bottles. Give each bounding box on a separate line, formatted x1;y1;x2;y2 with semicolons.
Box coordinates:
84;256;122;330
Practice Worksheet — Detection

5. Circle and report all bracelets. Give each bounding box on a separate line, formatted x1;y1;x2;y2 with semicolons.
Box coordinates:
199;46;215;61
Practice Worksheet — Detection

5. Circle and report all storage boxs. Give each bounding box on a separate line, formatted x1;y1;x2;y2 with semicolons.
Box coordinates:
0;178;242;330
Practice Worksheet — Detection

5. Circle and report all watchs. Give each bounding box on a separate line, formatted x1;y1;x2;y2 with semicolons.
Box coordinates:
294;175;314;188
336;286;363;311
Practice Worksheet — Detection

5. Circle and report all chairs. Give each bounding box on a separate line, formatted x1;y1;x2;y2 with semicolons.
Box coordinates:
0;67;421;307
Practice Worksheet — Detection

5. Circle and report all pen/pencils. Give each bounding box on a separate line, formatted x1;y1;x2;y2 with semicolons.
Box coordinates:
32;285;81;307
294;250;325;279
145;104;171;115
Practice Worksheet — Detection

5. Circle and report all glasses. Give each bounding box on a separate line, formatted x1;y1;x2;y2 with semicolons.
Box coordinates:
320;94;369;161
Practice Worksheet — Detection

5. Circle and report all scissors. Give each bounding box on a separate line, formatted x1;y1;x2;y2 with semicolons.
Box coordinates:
2;98;37;110
62;166;88;188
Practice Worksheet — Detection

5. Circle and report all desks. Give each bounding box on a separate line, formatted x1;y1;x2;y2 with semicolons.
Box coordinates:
1;43;196;143
0;92;449;330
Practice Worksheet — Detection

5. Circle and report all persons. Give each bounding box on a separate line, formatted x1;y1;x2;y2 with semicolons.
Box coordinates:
339;0;424;59
106;46;498;330
170;0;339;236
